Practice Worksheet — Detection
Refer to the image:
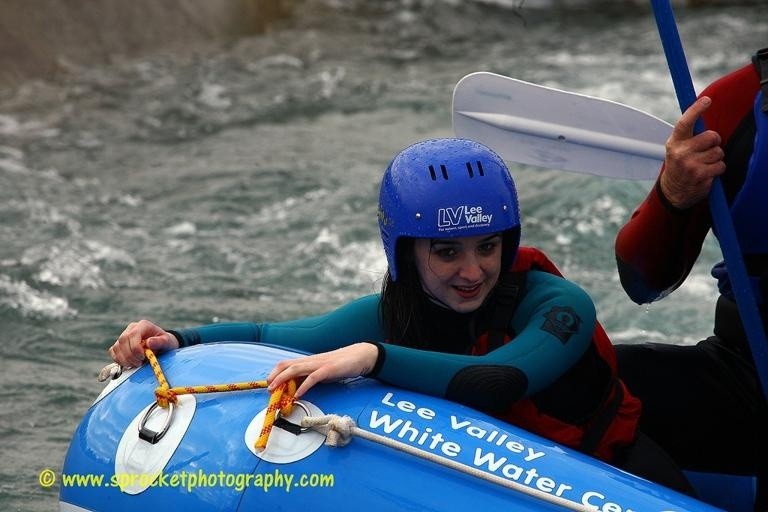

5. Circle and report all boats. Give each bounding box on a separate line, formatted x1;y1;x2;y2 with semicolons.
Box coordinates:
57;341;755;512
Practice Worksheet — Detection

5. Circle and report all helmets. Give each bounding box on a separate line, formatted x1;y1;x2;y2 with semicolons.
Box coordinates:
377;137;522;282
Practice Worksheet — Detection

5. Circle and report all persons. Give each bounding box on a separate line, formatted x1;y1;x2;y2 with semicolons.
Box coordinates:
106;138;700;497
615;48;766;512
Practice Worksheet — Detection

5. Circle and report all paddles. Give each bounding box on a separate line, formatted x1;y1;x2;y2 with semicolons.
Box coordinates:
452;71;675;179
